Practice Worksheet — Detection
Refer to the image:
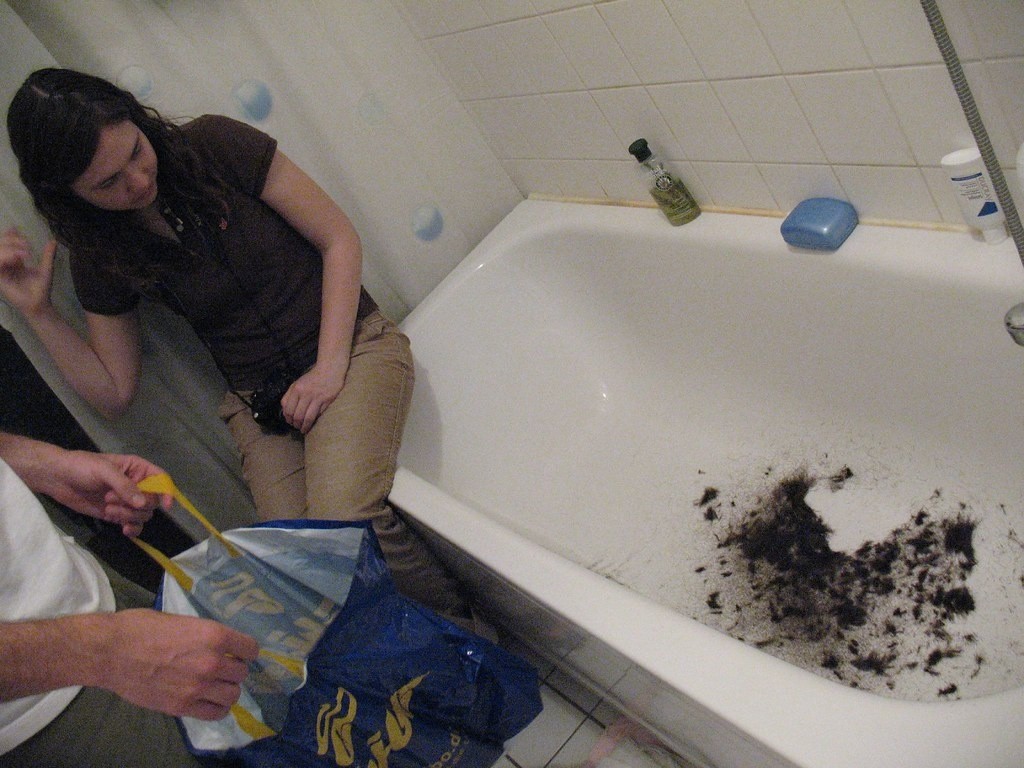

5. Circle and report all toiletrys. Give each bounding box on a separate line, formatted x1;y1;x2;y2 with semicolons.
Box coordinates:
941;148;1009;245
628;138;702;227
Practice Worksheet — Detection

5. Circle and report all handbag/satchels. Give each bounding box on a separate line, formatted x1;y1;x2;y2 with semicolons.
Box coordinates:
120;475;542;768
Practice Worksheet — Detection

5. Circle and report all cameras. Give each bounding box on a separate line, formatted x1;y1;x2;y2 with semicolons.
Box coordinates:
251;387;299;433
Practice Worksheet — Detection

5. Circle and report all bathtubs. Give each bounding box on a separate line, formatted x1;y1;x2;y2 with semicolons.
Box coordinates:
353;196;1023;768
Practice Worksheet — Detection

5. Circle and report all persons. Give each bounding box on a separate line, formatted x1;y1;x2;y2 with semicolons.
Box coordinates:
0;68;500;650
0;433;259;768
